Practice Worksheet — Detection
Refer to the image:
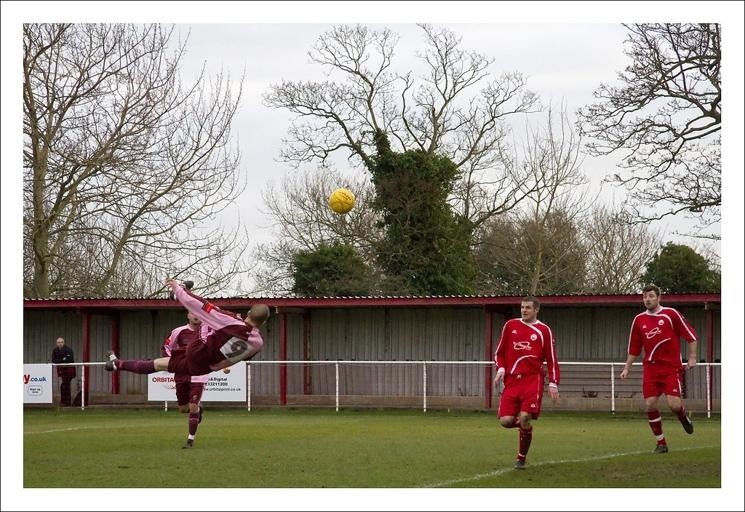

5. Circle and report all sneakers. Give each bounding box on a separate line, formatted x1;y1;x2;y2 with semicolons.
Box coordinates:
181;439;194;449
678;416;693;434
653;445;668;454
516;460;526;469
105;350;118;372
195;406;203;424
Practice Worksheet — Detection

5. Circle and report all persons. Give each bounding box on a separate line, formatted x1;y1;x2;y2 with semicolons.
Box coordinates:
104;278;271;376
52;337;77;407
493;295;561;470
621;285;701;454
161;310;231;449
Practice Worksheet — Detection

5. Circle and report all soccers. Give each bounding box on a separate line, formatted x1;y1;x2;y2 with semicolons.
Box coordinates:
330;189;356;213
